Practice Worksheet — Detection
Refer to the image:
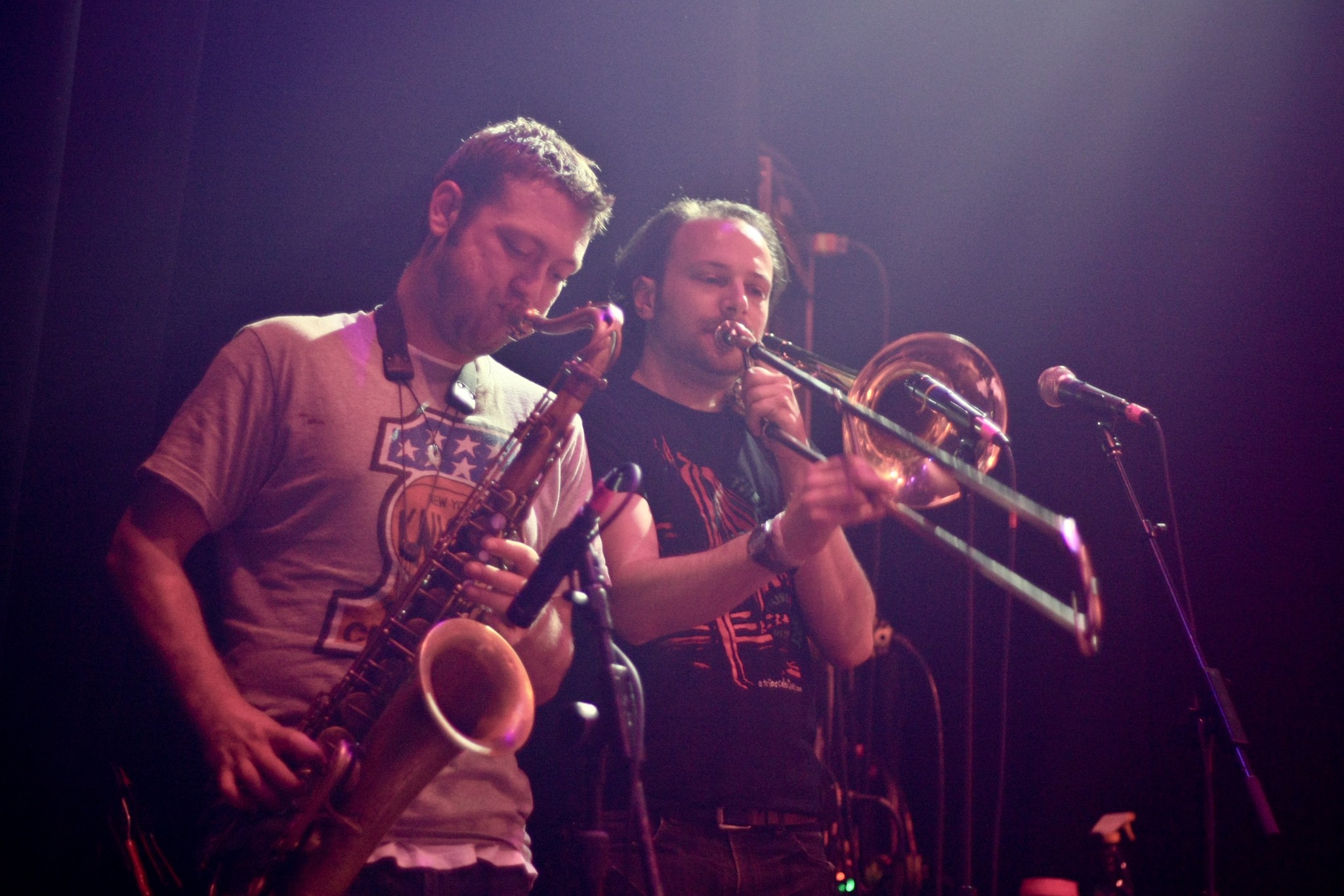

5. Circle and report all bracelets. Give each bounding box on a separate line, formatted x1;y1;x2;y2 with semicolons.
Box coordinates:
772;510;806;567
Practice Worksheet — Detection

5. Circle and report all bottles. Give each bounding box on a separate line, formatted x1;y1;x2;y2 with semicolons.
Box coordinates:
1021;878;1078;896
1094;832;1133;896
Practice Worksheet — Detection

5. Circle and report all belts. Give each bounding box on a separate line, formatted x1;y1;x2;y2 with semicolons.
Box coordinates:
572;802;818;830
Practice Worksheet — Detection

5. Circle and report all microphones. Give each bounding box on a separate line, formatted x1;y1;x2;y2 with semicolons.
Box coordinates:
1038;364;1156;427
502;466;623;628
904;370;1012;448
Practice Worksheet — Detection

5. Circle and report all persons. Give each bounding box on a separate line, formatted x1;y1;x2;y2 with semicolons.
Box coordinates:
581;194;899;896
107;116;614;896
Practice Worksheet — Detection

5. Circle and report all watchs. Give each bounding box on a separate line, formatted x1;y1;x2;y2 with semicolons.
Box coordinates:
744;513;802;575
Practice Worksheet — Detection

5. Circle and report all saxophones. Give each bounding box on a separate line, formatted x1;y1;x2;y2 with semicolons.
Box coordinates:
201;299;624;896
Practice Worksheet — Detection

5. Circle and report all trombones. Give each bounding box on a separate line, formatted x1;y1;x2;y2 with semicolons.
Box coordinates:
712;330;1102;659
